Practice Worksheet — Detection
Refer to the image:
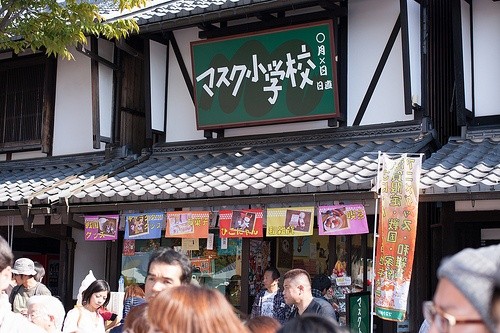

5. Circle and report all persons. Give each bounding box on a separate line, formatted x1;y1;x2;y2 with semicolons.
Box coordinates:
123;250;192;333
217;276;241;309
148;286;246;333
64;271;118;333
124;286;145;299
430;245;500;333
3;258;51;318
282;269;336;320
311;276;338;309
109;296;145;333
0;236;45;333
251;268;295;325
27;295;77;333
190;269;213;287
247;315;338;333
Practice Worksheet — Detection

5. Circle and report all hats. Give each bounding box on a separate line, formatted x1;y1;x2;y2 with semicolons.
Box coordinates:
10;258;37;276
437;243;500;333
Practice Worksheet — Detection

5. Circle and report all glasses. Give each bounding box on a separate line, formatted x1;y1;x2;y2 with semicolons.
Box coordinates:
423;303;484;333
